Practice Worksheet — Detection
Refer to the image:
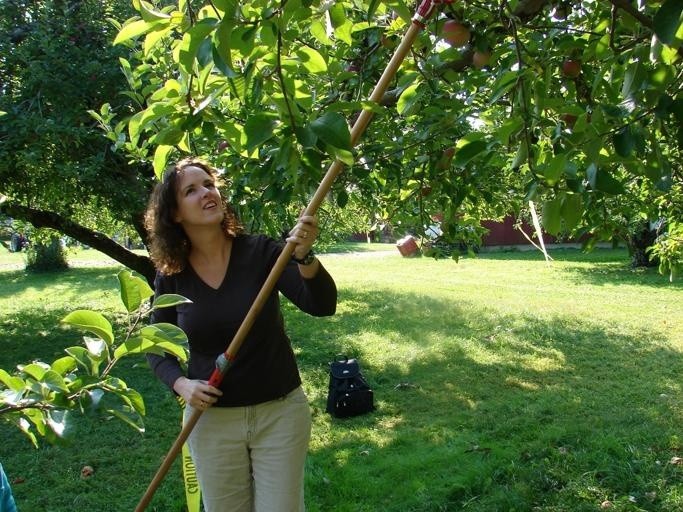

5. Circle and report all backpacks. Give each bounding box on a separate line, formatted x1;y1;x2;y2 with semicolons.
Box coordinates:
326;353;372;420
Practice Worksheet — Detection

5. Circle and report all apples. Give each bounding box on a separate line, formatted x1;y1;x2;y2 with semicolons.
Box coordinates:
442;19;471;48
554;4;572;19
563;114;576;123
473;51;492;68
423;186;433;195
444;148;455;156
218;141;231;152
348;112;360;128
560;61;580;78
345;65;361;71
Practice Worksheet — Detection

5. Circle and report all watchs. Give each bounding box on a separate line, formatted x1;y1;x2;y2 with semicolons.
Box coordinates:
292;251;315;266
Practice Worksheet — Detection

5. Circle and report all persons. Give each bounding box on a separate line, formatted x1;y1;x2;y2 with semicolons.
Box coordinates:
143;157;338;512
19;234;27;251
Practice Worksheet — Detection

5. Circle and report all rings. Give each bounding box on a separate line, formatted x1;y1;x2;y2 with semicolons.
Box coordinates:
303;230;308;240
200;400;208;408
